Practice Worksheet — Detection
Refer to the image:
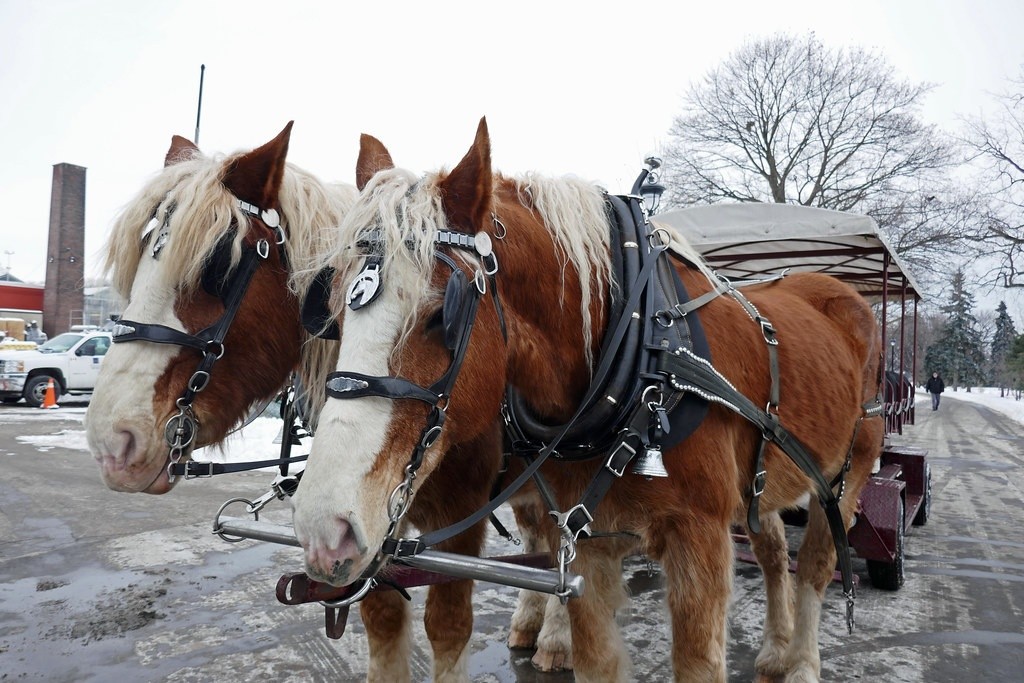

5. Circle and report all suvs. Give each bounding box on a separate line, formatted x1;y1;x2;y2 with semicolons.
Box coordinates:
0;328;112;406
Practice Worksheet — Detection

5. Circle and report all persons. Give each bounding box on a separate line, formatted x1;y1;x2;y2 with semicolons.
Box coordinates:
925;371;944;411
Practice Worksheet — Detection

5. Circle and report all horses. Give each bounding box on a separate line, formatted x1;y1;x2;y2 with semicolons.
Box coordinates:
286;114;886;683
86;118;574;683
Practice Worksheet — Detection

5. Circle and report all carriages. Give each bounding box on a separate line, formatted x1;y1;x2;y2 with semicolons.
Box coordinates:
91;122;934;680
